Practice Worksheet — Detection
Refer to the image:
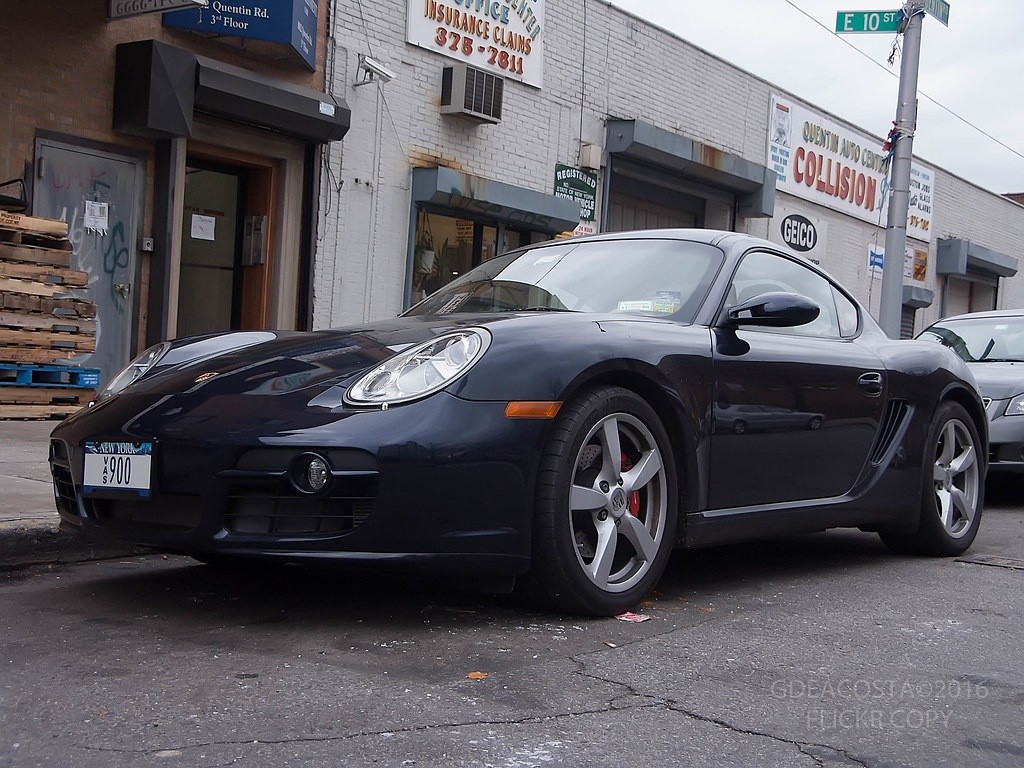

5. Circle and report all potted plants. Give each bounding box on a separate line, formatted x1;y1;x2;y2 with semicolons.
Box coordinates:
426;238;449;297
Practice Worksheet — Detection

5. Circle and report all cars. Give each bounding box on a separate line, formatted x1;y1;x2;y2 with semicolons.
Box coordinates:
918;308;1024;494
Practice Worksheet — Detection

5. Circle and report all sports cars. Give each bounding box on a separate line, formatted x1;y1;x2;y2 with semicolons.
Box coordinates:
44;225;994;620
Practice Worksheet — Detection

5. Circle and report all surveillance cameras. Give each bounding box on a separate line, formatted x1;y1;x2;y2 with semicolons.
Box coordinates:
360;56;396;83
